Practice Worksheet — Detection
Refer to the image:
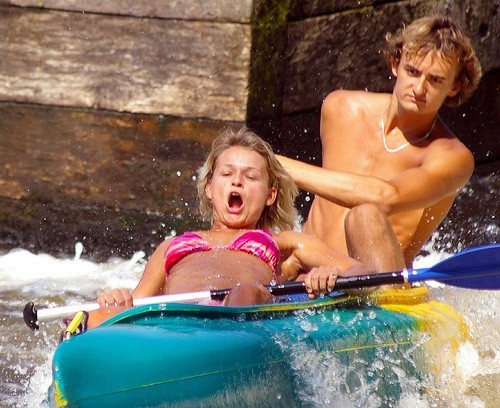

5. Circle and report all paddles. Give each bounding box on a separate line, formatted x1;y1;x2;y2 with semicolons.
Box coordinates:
23;245;500;331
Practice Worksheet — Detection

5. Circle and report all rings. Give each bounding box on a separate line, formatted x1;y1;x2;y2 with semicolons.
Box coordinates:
330;273;339;280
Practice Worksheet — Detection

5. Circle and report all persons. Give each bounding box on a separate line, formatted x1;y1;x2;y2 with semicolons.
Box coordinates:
274;14;485;289
87;121;381;328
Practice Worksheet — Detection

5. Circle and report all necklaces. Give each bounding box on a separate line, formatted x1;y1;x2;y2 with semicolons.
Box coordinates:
380;103;439;153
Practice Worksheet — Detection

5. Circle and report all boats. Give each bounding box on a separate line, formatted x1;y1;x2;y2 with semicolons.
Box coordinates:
53;286;474;408
